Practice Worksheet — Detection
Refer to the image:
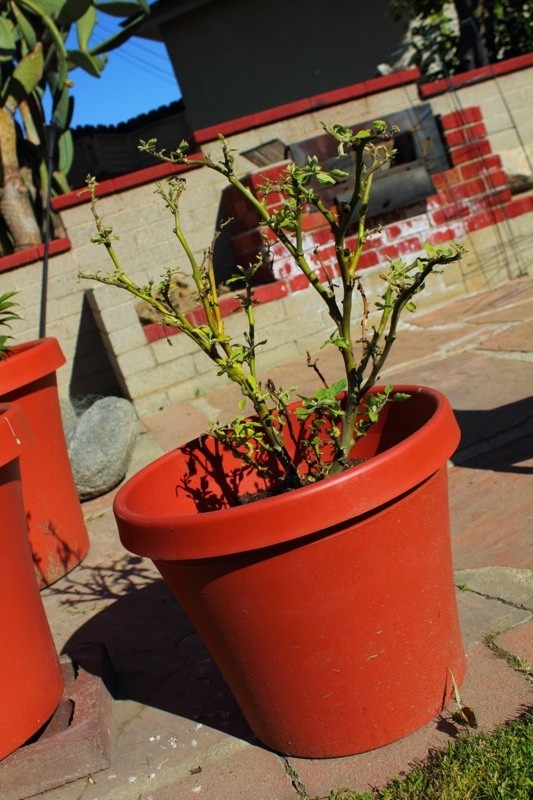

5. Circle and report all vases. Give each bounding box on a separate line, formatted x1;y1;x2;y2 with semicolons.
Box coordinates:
0;402;63;760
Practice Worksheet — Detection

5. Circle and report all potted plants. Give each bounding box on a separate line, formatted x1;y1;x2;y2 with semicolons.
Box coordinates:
0;291;89;590
78;115;465;756
